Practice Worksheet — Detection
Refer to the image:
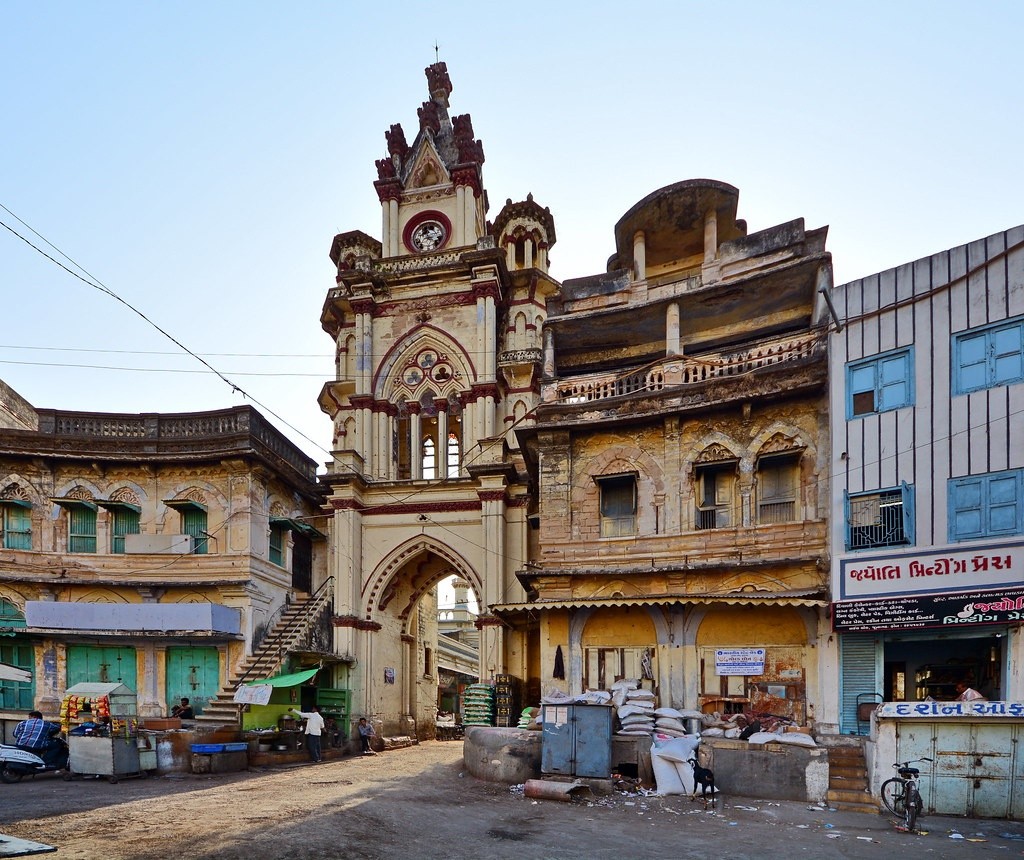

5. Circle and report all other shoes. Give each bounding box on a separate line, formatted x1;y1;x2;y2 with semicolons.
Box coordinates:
369;747;372;751
312;759;321;763
363;751;366;753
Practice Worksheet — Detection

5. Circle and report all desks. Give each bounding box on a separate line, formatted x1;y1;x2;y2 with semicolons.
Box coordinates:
248;730;301;753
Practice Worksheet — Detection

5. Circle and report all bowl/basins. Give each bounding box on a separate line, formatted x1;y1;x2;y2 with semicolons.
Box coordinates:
191;744;224;752
259;744;271;752
224;743;249;751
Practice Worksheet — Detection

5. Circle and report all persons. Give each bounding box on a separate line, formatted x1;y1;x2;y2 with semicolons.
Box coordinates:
953;676;990;703
166;697;195;718
358;718;376;751
287;705;324;762
14;711;61;767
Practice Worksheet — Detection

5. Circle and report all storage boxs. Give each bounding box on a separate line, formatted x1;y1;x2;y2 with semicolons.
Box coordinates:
190;742;249;753
144;719;182;731
494;674;521;727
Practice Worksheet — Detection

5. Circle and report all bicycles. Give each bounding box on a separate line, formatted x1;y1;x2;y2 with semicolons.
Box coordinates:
880;757;933;832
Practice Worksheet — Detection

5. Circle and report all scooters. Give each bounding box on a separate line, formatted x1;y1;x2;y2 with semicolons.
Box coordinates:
0;723;69;783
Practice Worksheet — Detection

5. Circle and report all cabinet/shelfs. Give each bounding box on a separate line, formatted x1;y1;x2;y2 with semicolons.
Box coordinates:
316;688;350;737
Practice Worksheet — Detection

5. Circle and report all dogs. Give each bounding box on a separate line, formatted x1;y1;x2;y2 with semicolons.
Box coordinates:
686;758;715;811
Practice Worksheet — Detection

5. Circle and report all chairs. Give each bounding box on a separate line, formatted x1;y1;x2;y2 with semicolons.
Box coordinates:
856;693;884;736
